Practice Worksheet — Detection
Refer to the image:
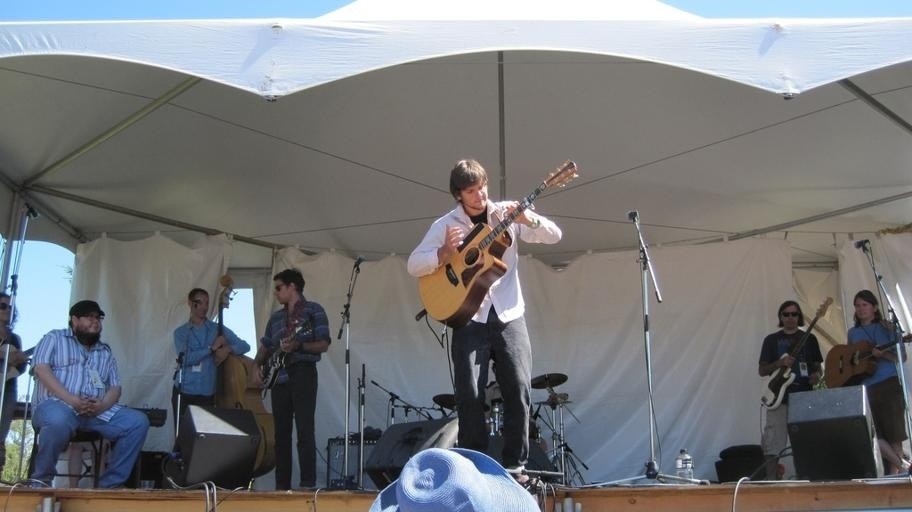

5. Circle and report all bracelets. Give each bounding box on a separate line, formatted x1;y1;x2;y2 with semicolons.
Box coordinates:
296;341;303;352
527;217;537;229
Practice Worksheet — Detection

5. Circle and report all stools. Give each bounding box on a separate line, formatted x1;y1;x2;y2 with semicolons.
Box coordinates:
28;428;115;489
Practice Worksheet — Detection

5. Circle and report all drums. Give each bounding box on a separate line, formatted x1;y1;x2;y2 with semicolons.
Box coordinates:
483;410;539;440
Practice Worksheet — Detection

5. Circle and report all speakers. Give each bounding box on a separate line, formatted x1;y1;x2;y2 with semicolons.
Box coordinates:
161;404;261;489
327;436;379;491
365;417;459;492
787;385;885;482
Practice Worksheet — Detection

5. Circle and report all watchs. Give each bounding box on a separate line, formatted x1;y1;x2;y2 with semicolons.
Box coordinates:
208;345;214;353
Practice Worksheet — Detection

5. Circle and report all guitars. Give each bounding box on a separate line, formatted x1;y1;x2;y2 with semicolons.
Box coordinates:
0;343;35;381
417;159;580;330
759;297;832;410
261;311;312;388
825;334;912;388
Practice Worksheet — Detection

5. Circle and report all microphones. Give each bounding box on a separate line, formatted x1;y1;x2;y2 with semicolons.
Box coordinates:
25;201;41;220
356;255;365;266
854;239;869;249
173;351;185;381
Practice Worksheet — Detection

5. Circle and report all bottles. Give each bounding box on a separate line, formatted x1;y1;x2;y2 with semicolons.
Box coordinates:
674;447;694;486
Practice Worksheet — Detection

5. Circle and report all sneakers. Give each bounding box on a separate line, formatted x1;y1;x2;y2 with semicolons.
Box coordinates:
512;472;540;487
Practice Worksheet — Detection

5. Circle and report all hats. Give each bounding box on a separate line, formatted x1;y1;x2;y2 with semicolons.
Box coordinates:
70;301;105;316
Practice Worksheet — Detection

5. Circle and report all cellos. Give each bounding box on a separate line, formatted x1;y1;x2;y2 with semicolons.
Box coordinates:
212;275;276;478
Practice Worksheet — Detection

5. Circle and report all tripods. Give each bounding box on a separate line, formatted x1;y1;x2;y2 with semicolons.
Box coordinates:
310;267;380;492
579;211;710;488
533;387;589;488
1;375;31;486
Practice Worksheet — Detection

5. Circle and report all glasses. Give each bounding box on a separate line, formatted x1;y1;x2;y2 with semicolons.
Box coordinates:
77;314;104;323
275;283;288;292
781;312;800;317
0;302;12;311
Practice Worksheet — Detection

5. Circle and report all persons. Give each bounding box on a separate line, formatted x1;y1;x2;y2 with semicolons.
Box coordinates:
407;158;562;483
28;299;153;489
172;287;250;443
847;290;912;478
251;268;332;490
0;292;27;484
758;300;824;481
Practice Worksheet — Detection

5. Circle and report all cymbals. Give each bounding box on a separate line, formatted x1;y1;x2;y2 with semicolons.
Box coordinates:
531;374;567;389
432;394;490;413
534;393;572;405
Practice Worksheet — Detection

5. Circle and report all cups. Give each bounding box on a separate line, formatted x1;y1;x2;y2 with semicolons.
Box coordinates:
136;478;155;490
